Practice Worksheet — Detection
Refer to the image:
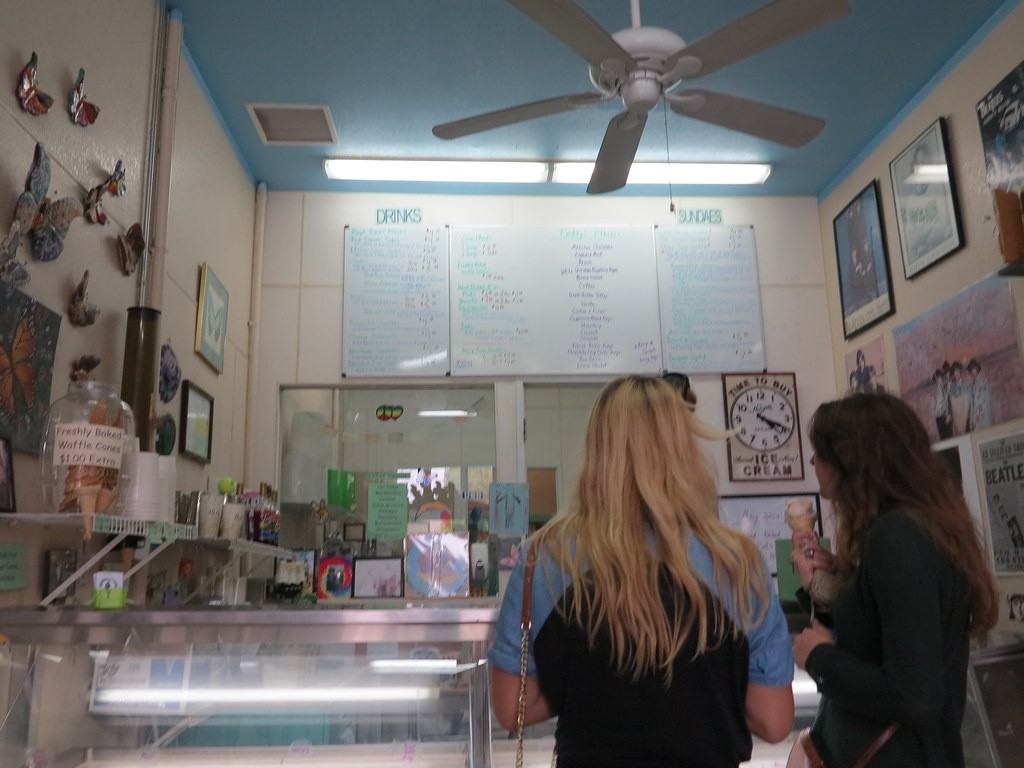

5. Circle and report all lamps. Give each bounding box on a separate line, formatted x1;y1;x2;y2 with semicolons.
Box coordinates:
323;156;772;186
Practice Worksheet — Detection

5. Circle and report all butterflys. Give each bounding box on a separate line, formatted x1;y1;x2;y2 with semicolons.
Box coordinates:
0;219;32;298
0;305;39;425
116;223;146;275
68;68;101;127
69;354;102;382
83;184;112;227
108;158;128;197
11;140;83;262
207;291;222;344
16;51;55;116
68;269;100;329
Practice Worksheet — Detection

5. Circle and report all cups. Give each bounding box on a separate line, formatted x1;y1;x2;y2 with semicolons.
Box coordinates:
126;452;179;524
220;503;246;538
199;495;225;538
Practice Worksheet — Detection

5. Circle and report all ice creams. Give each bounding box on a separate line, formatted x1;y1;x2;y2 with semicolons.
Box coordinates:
784;499;817;531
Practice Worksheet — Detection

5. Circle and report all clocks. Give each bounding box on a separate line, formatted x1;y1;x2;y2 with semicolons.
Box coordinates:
722;372;805;482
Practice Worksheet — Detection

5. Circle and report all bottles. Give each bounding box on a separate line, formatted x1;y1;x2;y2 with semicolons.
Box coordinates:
227;482;281;549
274;558;307;604
39;378;138;515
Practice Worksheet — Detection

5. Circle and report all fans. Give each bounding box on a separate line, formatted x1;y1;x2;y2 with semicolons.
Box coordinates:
432;0;851;194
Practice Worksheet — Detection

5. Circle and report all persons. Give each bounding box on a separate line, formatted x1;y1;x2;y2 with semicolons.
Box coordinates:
488;371;797;768
847;200;876;292
846;350;886;398
933;359;993;440
791;387;999;768
905;144;954;264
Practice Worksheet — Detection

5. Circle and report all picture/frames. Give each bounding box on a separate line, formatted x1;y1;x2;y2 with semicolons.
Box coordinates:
832;177;896;340
194;261;229;376
273;548;317;605
929;433;987;572
44;547;79;601
177;379;214;464
351;556;405;599
888;115;965;281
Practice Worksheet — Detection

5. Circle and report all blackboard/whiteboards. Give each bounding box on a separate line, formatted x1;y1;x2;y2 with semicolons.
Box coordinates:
342;225;768;378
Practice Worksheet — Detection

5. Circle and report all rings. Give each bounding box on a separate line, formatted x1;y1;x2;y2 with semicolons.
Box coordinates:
813;558;817;568
804;548;814;559
800;543;807;551
